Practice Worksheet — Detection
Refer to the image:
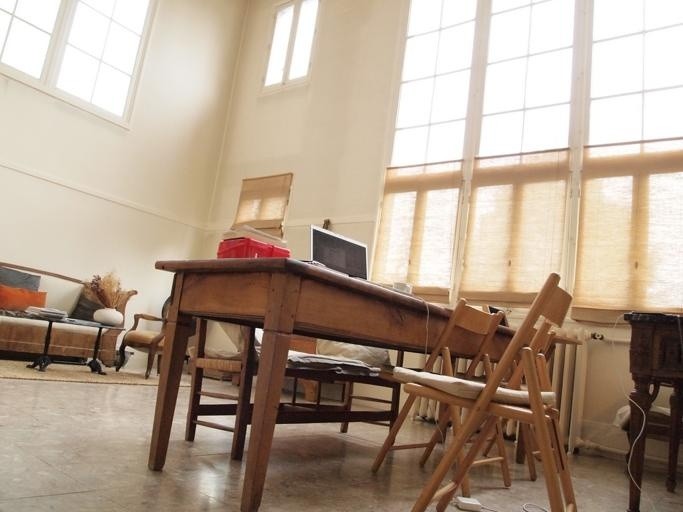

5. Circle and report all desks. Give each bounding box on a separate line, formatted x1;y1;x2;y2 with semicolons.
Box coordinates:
148;258;582;512
624;314;683;512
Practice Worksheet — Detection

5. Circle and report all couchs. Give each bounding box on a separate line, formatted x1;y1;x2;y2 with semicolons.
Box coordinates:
0;262;137;367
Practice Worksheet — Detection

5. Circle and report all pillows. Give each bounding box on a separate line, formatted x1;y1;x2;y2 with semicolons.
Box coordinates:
0;267;41;291
0;284;47;312
204;320;263;359
315;339;391;366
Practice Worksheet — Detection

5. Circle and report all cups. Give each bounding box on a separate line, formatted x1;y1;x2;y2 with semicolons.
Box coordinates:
393;282;413;294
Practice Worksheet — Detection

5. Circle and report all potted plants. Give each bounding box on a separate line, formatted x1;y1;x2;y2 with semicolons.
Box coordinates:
84;274;129;326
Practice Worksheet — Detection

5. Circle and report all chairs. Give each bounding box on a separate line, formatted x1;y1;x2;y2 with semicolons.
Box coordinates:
371;299;512;498
612;405;683;476
482;329;558;482
116;296;171;379
393;273;577;512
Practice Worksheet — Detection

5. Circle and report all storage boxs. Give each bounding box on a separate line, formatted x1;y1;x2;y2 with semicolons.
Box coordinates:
217;237;290;258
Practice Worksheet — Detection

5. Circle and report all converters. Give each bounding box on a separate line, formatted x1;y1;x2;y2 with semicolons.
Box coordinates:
454;496;482;512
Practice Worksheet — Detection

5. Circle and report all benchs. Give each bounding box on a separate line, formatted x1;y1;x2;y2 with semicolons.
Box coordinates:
185;317;404;460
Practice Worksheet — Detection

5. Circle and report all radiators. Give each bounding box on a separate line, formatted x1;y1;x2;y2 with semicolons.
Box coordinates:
407;321;606;456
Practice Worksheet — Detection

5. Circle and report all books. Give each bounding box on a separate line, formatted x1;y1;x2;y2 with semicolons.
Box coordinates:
24;304;69;319
220;224;289;250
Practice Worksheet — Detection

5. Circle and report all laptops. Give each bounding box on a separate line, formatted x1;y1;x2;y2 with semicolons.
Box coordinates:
310;224;368;280
